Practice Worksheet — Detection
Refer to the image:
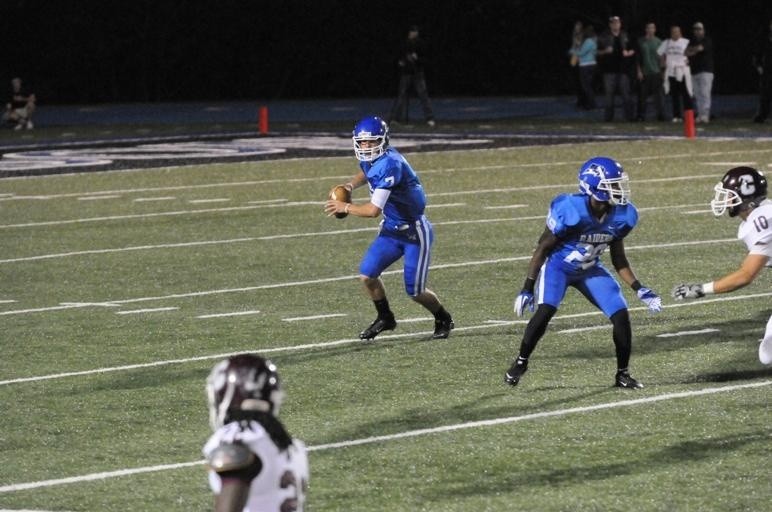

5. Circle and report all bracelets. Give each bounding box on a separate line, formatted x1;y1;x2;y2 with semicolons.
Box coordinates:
346;184;354;191
342;202;353;214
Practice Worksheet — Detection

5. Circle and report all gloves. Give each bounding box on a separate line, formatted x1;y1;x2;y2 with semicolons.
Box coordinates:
670;282;707;302
513;289;536;315
637;286;663;312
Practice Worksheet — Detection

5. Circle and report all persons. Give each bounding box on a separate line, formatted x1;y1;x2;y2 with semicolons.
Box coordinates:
670;162;772;367
568;18;713;123
751;35;772;122
502;156;660;387
390;26;435;126
322;115;455;342
4;75;40;133
197;351;308;511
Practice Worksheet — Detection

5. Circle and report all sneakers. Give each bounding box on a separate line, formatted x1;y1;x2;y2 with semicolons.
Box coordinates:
357;312;397;341
502;356;528;388
431;316;455;340
615;372;643;388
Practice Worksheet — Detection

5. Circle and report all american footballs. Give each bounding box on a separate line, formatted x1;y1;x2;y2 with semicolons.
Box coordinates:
329;187;350;220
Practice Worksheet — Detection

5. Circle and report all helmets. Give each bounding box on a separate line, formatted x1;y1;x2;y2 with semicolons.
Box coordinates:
578;156;631;206
205;353;281;431
352;115;390;161
710;166;767;219
